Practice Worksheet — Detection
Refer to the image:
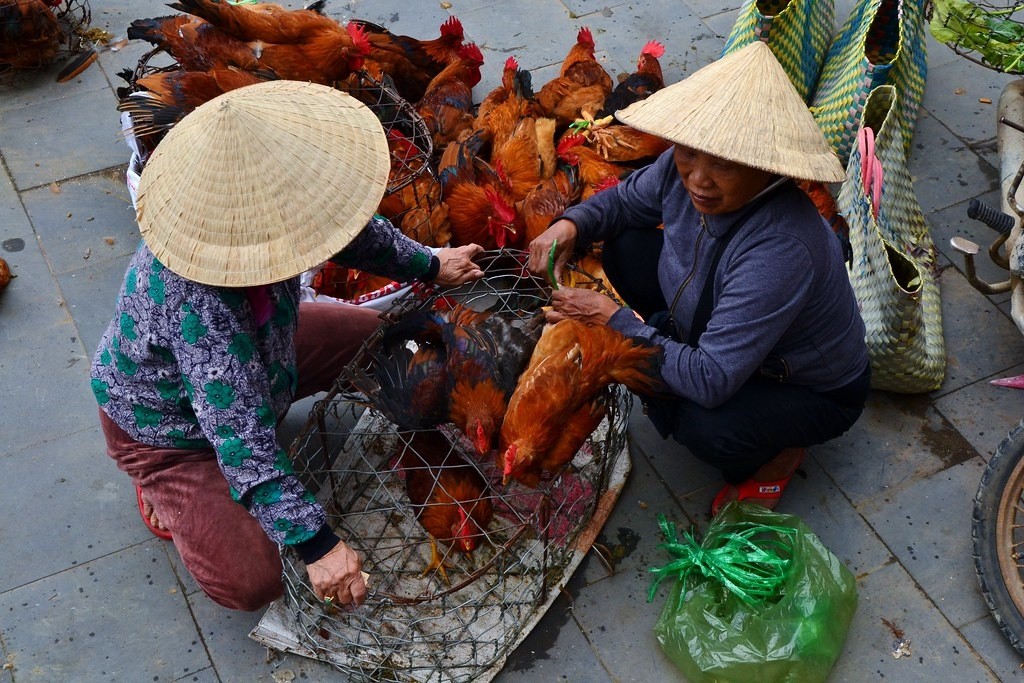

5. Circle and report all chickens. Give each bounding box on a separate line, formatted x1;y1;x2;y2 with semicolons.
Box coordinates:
113;0;855;582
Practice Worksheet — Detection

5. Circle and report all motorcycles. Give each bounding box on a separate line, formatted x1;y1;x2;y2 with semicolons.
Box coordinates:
950;80;1024;658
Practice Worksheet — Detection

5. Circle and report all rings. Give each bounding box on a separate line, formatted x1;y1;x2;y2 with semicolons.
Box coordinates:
324;594;335;605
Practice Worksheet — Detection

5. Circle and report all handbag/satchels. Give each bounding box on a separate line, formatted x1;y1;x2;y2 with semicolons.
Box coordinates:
725;0;945;392
652;499;858;683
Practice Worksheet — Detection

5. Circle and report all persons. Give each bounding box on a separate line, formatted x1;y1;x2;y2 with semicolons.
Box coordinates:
526;37;875;516
86;78;487;611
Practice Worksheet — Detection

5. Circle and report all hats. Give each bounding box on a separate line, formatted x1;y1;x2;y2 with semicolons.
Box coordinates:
135;81;390;287
615;41;846;182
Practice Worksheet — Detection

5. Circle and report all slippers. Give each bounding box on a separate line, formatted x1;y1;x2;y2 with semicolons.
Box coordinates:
711;444;805;517
135;482;172;541
